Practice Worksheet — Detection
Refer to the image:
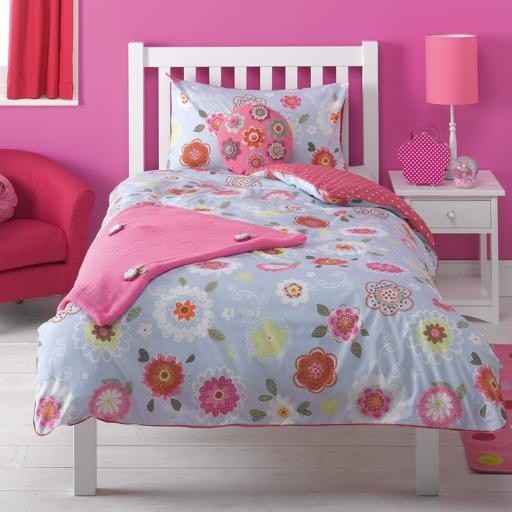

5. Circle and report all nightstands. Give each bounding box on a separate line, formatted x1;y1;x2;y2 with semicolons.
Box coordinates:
387;167;505;325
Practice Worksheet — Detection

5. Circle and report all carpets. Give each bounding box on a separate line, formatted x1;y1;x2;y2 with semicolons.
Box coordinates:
461;344;512;475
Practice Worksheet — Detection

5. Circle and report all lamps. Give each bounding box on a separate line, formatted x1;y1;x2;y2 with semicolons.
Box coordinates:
424;35;479;180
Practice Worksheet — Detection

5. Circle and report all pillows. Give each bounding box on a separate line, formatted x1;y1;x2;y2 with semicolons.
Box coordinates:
160;76;353;175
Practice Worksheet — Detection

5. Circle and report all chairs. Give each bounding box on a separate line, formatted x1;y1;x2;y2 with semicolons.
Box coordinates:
1;148;96;305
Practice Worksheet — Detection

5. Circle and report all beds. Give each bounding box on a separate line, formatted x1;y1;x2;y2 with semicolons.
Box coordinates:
30;40;506;497
